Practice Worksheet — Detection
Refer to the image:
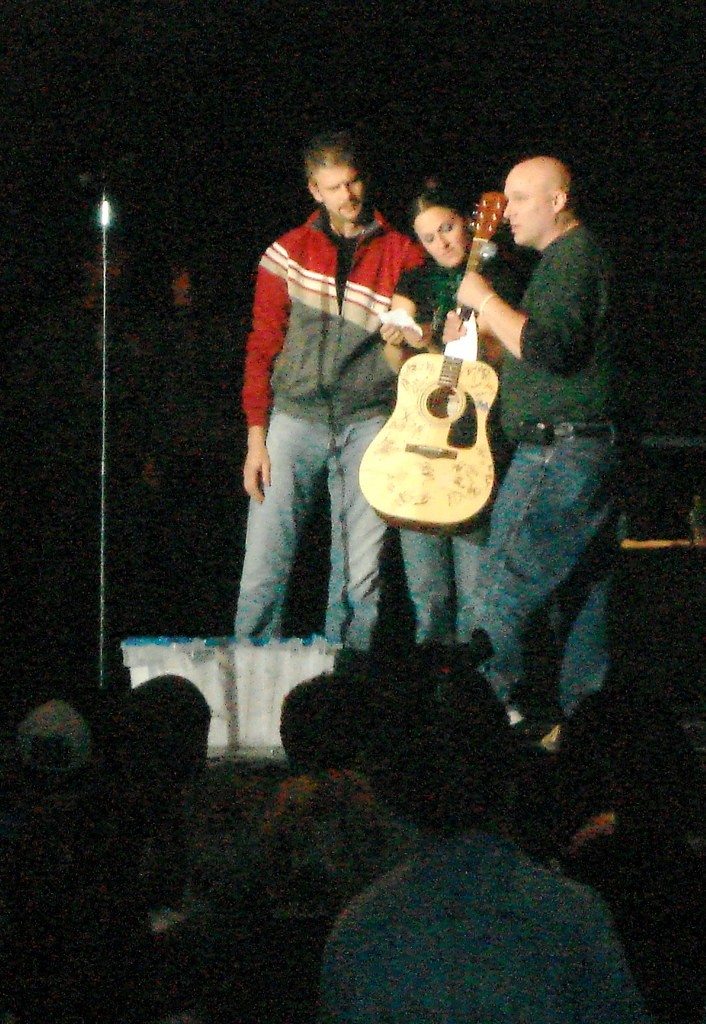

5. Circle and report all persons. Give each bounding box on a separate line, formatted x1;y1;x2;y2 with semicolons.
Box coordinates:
233;130;623;714
0;640;706;1024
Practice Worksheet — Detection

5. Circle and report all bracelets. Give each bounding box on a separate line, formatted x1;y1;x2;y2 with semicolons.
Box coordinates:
480;291;499;317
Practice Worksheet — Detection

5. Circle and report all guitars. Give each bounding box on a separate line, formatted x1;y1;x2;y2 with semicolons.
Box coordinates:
360;191;508;530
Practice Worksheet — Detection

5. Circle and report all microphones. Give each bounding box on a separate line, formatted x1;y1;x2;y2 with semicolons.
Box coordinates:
458;242;497;331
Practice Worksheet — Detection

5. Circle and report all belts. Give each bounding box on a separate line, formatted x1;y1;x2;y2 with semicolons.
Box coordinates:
516;423;620;445
